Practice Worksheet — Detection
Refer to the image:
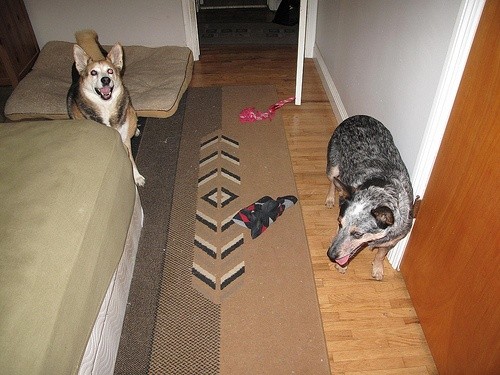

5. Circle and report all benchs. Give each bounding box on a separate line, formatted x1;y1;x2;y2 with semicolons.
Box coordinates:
0;120;146;375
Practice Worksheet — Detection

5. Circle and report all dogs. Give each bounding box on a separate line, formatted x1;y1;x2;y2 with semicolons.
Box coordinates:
325;111;418;281
68;41;150;190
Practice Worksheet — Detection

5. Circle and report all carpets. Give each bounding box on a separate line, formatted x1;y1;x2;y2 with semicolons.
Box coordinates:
113;84;331;375
198;21;299;45
